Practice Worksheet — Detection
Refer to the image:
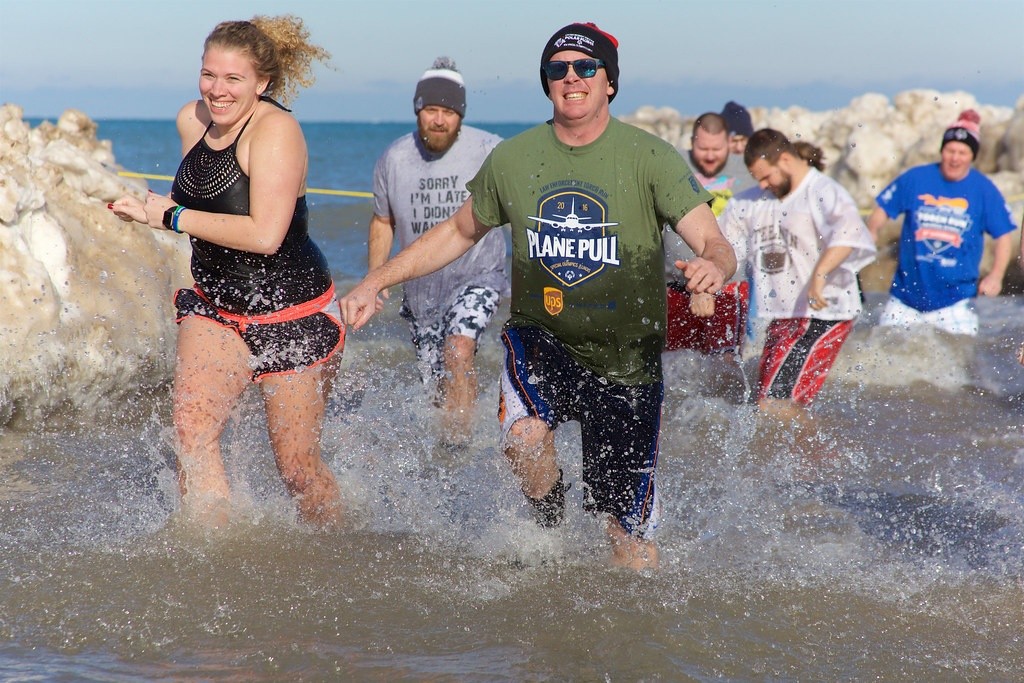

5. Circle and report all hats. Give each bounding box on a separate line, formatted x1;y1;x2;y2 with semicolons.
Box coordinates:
940;110;981;160
539;23;619;104
413;56;466;116
719;102;752;137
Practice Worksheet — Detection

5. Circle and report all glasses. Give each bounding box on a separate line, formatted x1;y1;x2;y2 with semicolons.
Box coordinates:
541;60;605;80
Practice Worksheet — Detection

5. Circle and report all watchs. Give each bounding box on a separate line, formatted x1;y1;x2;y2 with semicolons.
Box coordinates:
163;205;178;231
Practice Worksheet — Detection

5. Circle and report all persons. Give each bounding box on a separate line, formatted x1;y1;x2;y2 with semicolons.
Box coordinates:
786;141;869;307
711;104;753;160
336;25;736;576
106;16;344;530
362;55;512;456
867;108;1020;343
731;126;882;472
658;110;758;378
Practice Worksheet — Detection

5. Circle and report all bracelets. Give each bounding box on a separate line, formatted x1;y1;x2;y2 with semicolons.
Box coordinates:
173;205;186;234
